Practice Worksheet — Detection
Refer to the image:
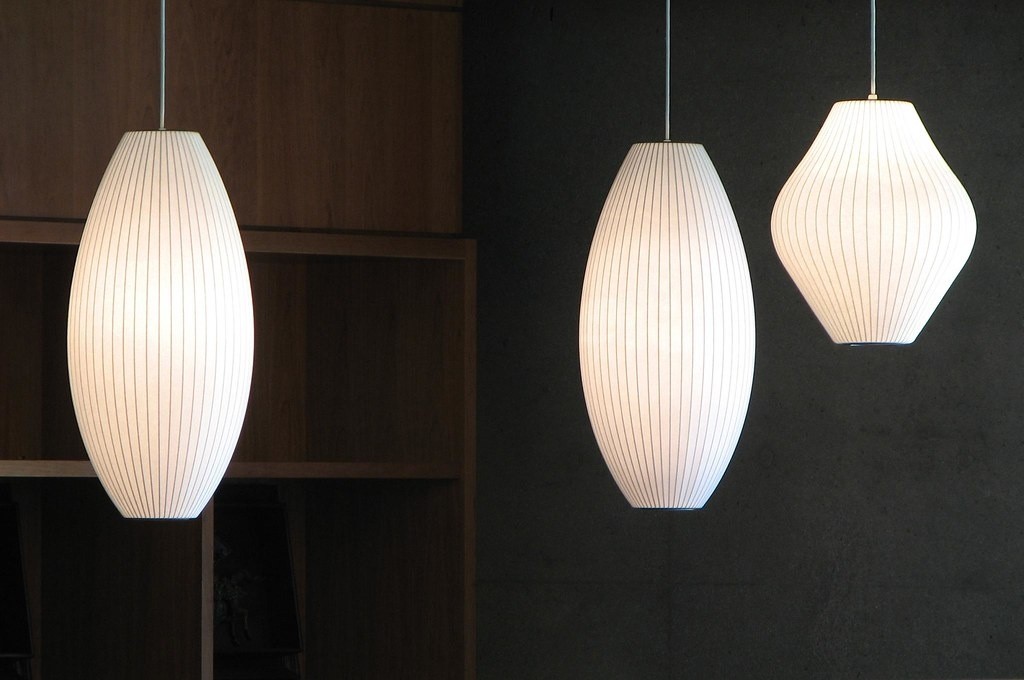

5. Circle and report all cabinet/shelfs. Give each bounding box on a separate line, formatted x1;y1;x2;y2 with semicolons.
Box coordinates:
0;0;478;680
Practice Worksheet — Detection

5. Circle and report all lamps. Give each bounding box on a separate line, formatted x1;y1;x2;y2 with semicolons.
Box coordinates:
579;0;757;510
66;0;255;519
770;0;977;347
